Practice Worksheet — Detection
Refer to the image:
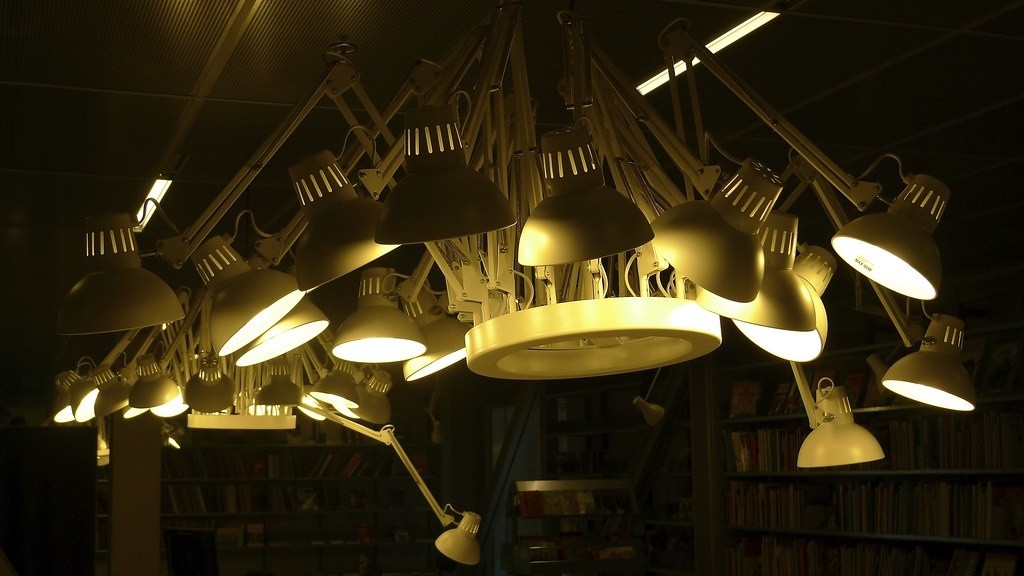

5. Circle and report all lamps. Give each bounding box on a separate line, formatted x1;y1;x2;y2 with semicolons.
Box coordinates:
55;0;982;576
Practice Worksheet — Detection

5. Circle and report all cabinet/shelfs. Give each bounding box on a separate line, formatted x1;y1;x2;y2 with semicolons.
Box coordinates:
690;3;1024;576
540;368;700;576
160;403;494;576
500;476;654;576
94;451;113;565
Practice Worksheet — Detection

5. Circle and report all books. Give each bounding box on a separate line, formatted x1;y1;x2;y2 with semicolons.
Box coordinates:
516;488;635;517
161;421;444;576
94;462;112;565
517;518;696;576
719;337;1024;576
